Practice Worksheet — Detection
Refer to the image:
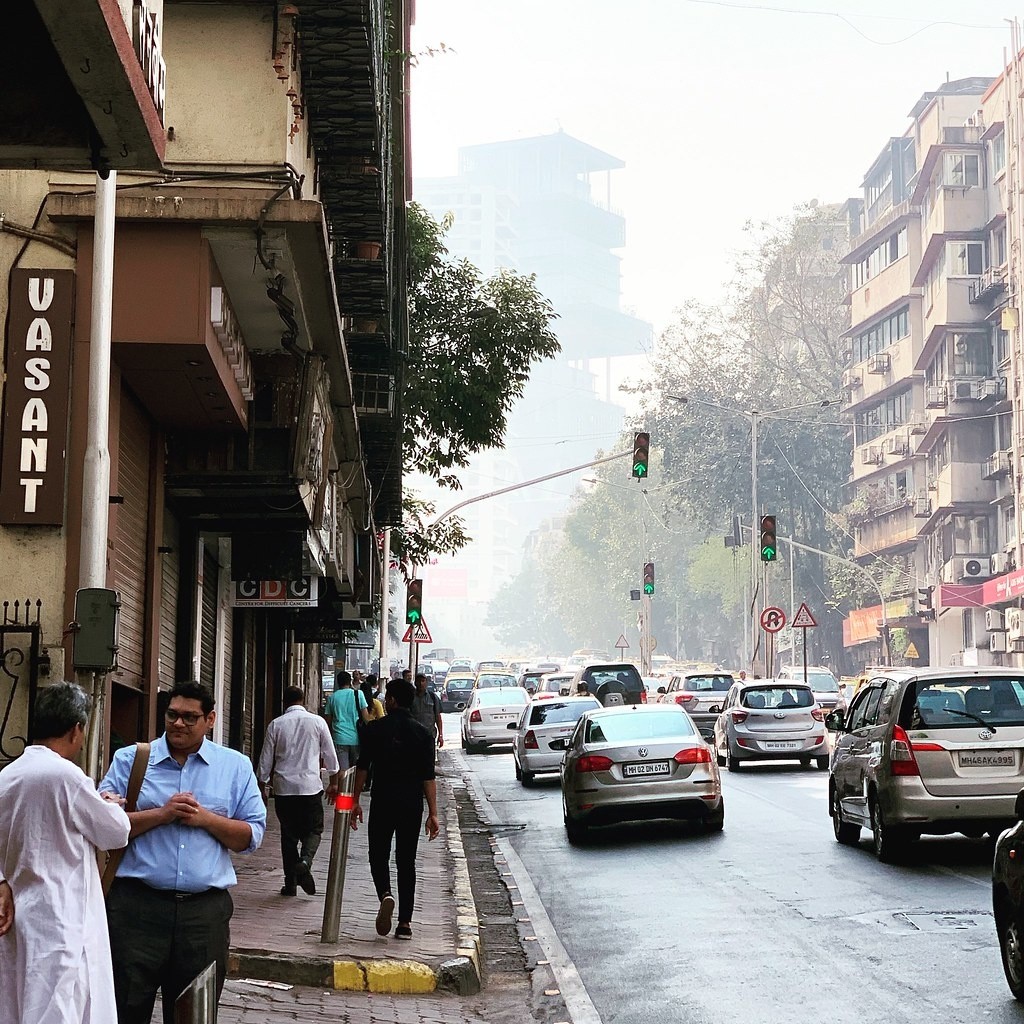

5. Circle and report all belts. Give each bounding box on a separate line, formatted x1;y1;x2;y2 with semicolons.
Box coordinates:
125;879;224;902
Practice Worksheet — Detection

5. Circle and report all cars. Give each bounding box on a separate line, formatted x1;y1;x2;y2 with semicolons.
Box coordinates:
533;673;574;699
547;704;725;838
475;660;561;692
708;679;830;773
392;657;475;701
457;686;532;754
992;788;1024;1003
638;677;664;705
506;697;605;786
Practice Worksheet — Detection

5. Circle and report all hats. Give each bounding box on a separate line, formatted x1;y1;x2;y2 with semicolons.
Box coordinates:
416;673;426;680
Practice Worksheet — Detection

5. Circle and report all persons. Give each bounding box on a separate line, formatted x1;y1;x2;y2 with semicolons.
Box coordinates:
0;678;132;1024
412;673;444;748
366;674;387;715
754;675;763;679
350;669;363;690
349;678;440;941
94;681;267;1024
738;670;752;681
570;681;594;696
360;682;386;723
256;685;340;896
394;672;400;678
402;670;412;682
387;670;393;683
325;671;374;763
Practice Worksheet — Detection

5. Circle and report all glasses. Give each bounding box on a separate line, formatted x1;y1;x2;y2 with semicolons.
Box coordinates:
165;711;207;726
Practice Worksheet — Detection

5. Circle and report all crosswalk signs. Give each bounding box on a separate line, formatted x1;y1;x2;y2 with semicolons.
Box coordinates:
402;617;432;643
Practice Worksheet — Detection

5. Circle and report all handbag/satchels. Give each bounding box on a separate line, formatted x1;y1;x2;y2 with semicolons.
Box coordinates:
357;718;366;740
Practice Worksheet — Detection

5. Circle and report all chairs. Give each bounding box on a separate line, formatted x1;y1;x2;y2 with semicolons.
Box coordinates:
450;683;457;689
482;679;491;688
970;689;995;716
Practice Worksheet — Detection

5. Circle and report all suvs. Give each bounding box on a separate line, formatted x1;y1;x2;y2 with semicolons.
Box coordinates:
777;666;847;718
568;663;647;708
825;666;1024;861
658;671;737;736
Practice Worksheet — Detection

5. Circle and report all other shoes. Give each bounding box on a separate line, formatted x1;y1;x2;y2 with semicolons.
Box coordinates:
280;886;297;896
296;862;317;894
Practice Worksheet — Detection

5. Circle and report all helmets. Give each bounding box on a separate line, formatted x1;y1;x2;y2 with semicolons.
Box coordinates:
577;681;588;693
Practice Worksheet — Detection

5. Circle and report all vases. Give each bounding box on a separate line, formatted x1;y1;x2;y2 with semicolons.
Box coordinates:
356;321;377;332
356;241;382;259
351;156;370;173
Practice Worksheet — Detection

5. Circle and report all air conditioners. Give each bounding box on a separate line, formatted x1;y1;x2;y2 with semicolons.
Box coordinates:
986;607;1024;653
963;558;990;577
981;451;1009;480
843;369;863;388
911;414;924;435
981;380;995;400
953;380;979;401
887;435;904;454
914;498;925;518
861;446;877;464
868;354;884;372
991;552;1008;575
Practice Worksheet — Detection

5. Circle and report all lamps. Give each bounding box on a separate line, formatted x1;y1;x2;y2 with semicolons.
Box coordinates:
266;272;307;364
927;387;937;407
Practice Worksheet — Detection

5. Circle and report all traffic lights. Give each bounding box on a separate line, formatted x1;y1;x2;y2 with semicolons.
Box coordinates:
406;578;422;624
633;431;650;478
918;587;932;608
644;562;655;594
760;515;777;561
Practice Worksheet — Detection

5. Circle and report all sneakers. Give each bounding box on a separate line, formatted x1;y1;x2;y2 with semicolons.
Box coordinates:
394;926;412;940
375;893;396;936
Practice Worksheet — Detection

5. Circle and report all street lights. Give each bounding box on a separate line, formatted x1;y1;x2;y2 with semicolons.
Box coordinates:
666;392;841;679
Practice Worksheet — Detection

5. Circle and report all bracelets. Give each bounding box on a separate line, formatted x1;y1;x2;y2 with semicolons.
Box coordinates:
438;735;443;737
